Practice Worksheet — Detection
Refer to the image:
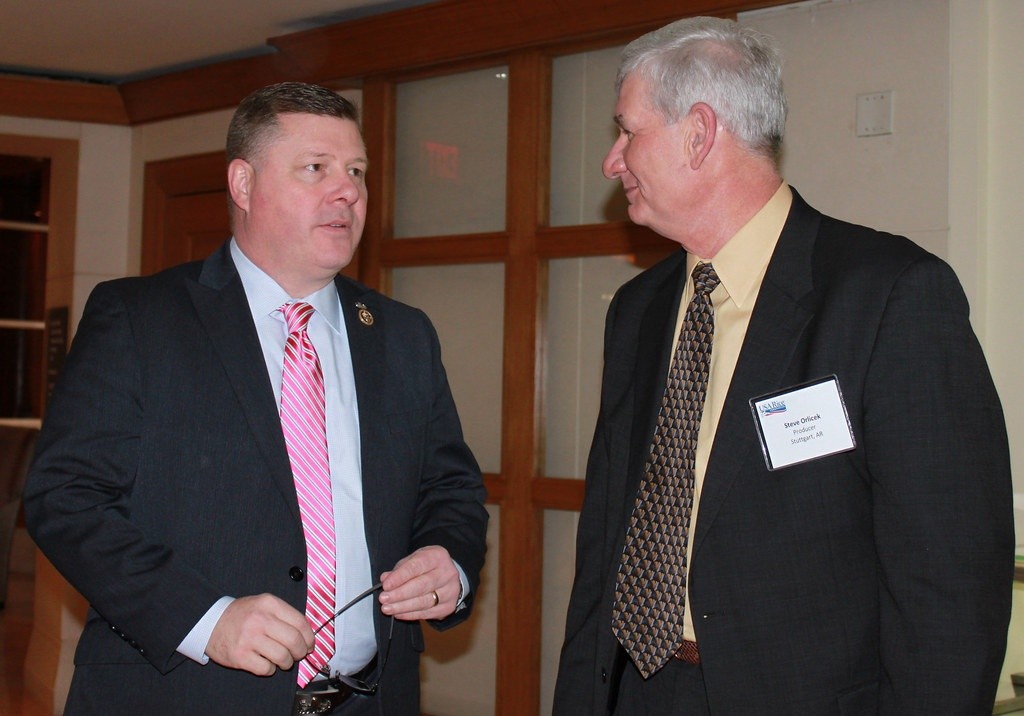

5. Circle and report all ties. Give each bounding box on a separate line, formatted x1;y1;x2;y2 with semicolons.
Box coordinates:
612;265;722;680
280;298;336;689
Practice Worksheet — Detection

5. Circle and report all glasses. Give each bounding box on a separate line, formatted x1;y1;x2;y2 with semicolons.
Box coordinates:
304;583;394;695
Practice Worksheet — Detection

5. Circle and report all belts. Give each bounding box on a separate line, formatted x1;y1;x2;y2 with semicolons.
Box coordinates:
294;653;378;714
670;640;702;666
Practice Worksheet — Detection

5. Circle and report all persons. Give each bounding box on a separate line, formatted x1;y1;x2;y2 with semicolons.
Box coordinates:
550;13;1018;716
20;78;491;716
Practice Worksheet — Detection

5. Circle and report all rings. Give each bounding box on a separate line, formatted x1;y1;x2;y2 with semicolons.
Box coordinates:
432;590;440;607
